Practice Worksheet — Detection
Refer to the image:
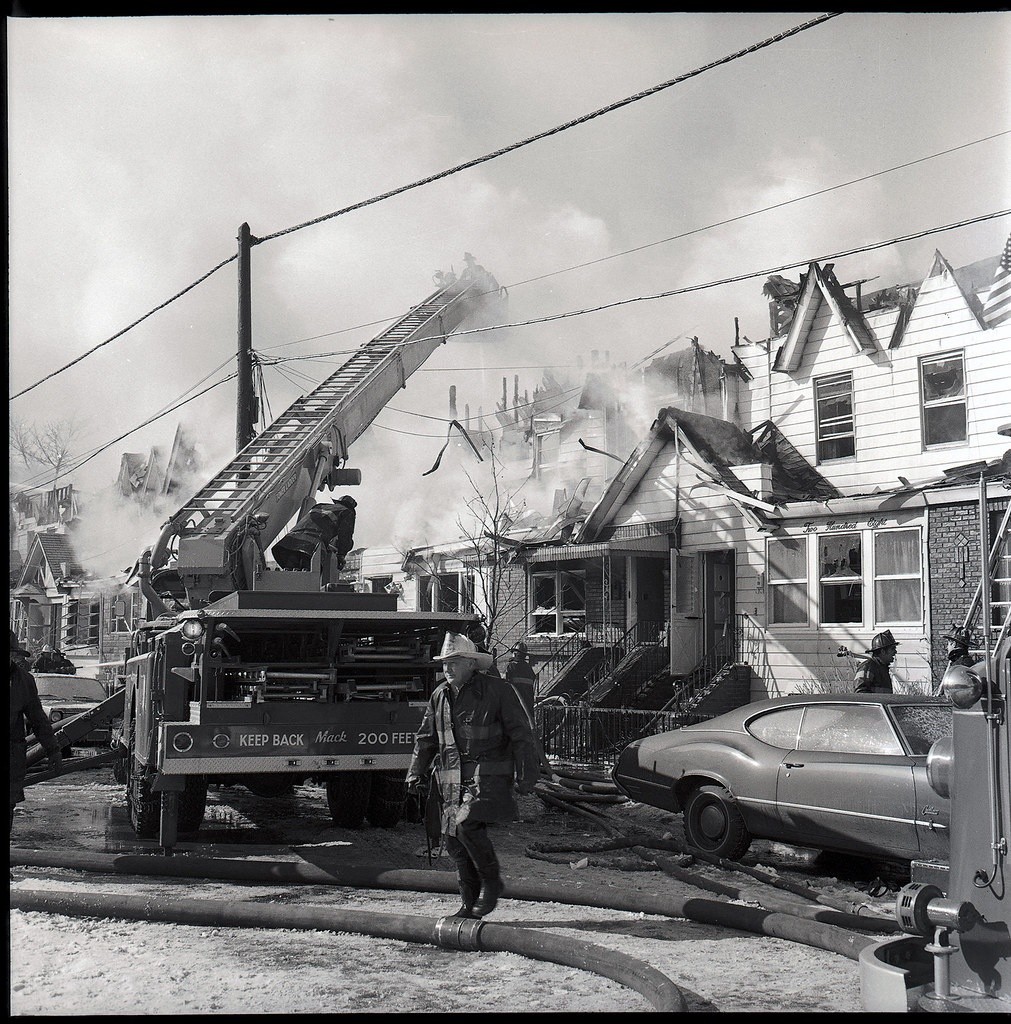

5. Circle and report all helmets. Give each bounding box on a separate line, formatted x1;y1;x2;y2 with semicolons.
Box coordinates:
338;495;357;508
865;630;899;653
42;644;54;653
943;627;977;646
53;649;65;655
510;642;530;655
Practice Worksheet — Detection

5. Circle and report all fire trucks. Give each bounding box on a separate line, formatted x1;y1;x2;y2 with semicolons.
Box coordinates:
110;252;510;849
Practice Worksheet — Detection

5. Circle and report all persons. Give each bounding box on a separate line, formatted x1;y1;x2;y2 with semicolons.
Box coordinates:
271;495;357;571
8;629;62;880
35;645;76;675
405;631;539;920
854;633;899;694
943;626;978;667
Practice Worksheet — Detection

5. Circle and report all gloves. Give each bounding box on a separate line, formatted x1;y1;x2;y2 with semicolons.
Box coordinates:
515;784;533;796
337;555;346;571
48;751;62;774
408;783;418;794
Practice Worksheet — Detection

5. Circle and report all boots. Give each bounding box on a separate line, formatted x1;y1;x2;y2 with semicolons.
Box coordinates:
466;838;505;917
452;861;484;920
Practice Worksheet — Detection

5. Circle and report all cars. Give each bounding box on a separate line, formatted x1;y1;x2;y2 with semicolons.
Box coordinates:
613;694;983;868
24;672;112;747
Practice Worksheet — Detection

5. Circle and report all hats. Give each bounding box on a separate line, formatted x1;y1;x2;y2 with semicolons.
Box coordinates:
433;630;493;671
10;630;31;657
